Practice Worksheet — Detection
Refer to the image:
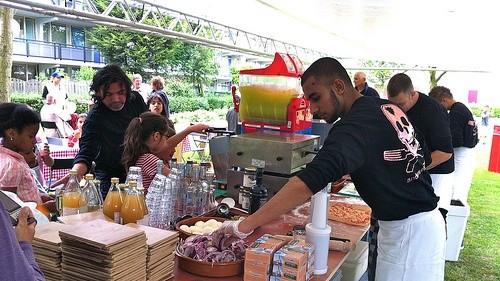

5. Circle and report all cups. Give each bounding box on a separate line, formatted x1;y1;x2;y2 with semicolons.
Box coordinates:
124;166;144;195
35;132;48;157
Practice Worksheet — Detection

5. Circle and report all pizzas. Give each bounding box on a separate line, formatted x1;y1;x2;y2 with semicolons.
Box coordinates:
327;203;372;227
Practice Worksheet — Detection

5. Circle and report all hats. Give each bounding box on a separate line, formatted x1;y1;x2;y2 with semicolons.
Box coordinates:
52;72;64;78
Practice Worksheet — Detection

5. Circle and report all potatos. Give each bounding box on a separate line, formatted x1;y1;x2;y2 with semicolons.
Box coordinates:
180;218;224;235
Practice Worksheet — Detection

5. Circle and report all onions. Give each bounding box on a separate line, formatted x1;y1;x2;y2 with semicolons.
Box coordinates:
180;233;249;264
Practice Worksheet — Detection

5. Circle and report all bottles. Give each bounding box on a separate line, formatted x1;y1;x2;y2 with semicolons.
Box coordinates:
156;157;215;218
60;170;148;225
249;166;268;215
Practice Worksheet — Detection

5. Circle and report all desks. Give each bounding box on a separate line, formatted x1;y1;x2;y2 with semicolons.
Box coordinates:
164;173;372;281
39;138;80;180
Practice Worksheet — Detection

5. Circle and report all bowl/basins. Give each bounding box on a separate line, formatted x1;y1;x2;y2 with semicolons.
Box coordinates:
178;242;245;277
176;216;230;241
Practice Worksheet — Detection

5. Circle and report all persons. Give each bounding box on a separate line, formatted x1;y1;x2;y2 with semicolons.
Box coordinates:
481;105;491;145
40;93;71;138
41;72;68;110
226;95;240;131
387;73;455;222
0;200;46;281
354;72;380;98
428;86;480;249
50;65;152;194
0;102;58;221
120;74;209;197
71;113;87;138
209;57;447;281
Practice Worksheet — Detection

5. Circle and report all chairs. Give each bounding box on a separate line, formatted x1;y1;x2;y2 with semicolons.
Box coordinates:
41;120;65;139
48;158;75;192
190;123;229;162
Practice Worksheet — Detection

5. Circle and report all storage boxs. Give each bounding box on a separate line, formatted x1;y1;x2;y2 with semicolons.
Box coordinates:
446;200;470;261
243;234;316;281
339;241;369;280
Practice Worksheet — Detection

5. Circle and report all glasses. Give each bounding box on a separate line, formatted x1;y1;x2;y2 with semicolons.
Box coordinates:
161;133;170;139
